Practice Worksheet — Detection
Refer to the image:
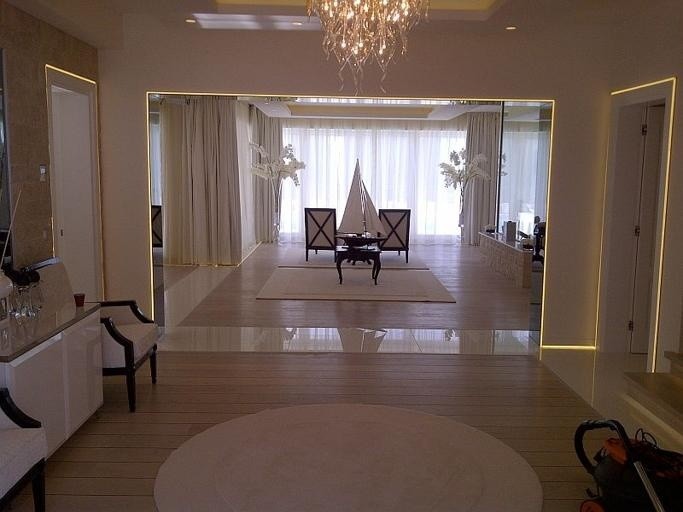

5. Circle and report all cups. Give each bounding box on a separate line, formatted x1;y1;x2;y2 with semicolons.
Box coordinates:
73;293;86;307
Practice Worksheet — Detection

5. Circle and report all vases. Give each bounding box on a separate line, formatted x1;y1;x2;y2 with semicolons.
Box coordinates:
269;211;281;246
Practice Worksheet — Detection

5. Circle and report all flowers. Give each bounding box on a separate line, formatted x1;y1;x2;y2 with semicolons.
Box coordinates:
438;146;492;215
249;141;304;211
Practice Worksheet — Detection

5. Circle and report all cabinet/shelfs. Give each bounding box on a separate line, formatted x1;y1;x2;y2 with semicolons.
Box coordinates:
0;308;103;499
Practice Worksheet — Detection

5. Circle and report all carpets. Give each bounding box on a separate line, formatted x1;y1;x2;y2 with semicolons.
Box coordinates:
254;244;456;303
153;403;544;511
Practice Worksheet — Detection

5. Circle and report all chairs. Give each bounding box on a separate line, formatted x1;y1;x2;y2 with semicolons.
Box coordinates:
377;209;410;263
304;208;336;262
17;261;160;414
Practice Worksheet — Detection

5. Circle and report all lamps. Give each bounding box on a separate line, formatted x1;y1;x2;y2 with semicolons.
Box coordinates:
300;1;434;105
